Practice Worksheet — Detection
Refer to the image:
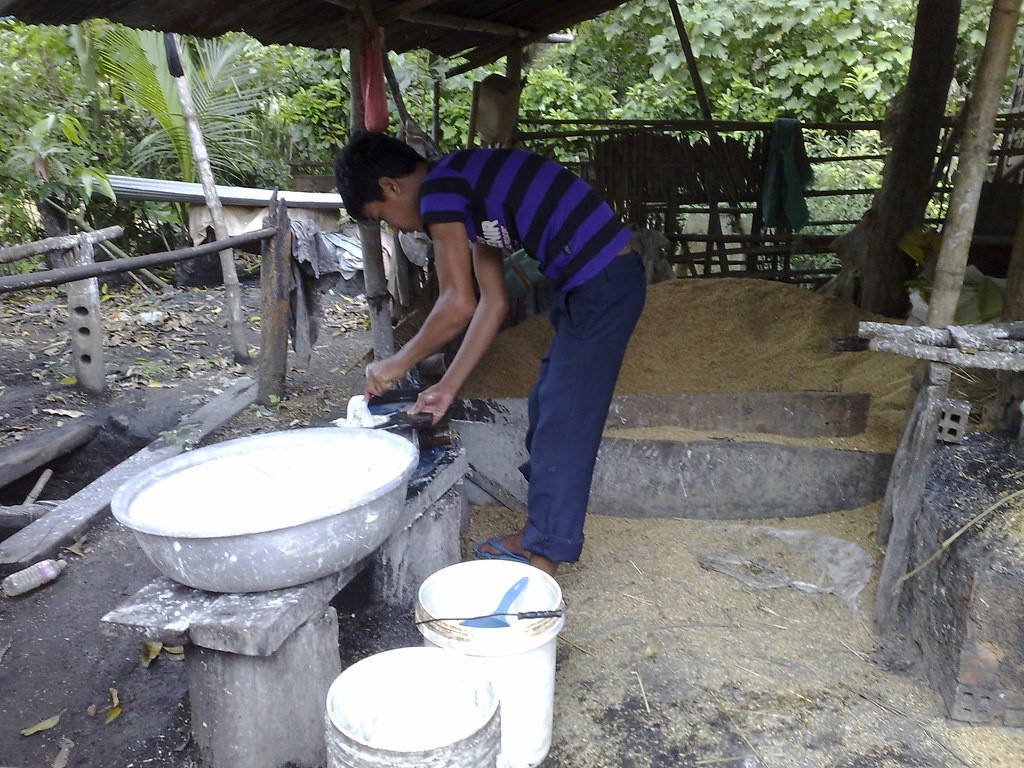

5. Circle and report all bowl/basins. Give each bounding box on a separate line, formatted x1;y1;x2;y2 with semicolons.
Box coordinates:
110;427;419;593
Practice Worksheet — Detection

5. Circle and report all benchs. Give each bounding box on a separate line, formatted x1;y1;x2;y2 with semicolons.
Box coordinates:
98;448;471;768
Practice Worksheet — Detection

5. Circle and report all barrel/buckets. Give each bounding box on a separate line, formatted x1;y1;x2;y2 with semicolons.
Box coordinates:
324;646;502;768
414;558;566;768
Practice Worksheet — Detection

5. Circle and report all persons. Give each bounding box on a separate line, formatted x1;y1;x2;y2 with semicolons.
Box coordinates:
332;125;647;582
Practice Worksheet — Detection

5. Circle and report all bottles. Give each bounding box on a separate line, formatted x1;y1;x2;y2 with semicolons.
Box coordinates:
2;559;68;597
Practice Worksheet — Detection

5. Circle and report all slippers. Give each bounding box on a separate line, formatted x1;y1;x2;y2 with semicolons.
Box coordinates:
472;536;530;564
